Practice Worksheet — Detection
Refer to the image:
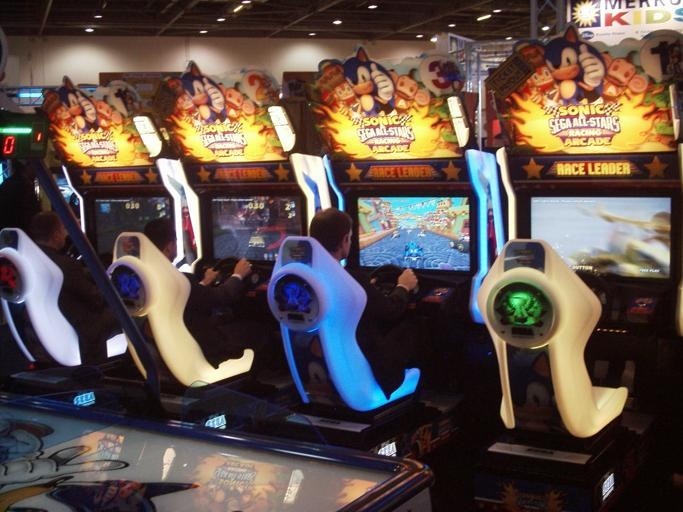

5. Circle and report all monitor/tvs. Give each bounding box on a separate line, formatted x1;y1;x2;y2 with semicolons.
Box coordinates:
358;196;470;271
531;197;672;277
210;196;298;266
96;198;176;267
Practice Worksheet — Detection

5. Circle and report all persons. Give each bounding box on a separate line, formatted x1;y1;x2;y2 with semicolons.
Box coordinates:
23;211;118;345
303;210;417;386
142;219;253;372
